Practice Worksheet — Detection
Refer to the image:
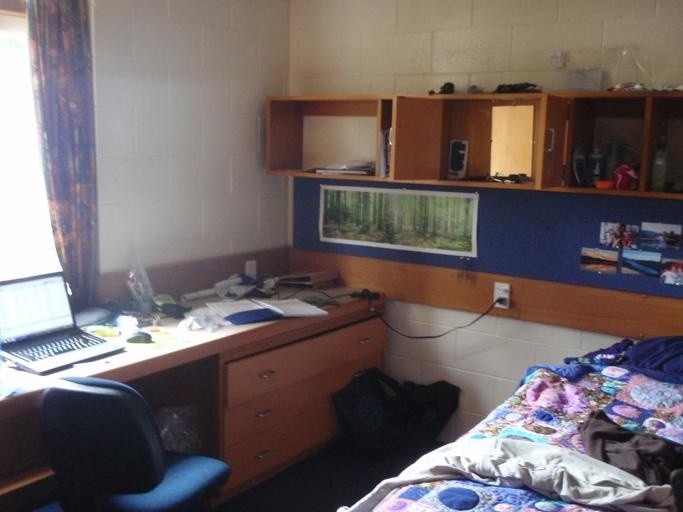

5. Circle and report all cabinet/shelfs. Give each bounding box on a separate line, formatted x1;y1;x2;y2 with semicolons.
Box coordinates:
221;315;389;494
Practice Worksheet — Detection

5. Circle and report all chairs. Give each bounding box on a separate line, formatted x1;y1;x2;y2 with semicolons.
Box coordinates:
40;374;232;510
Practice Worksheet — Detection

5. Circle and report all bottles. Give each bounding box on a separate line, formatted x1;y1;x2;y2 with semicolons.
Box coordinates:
649;134;668;193
587;148;603;182
603;46;647;92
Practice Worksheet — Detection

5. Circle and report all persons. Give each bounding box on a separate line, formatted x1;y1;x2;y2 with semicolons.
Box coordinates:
598;216;683;287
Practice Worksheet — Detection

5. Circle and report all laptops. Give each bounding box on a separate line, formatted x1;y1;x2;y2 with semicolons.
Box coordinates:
0;272;125;375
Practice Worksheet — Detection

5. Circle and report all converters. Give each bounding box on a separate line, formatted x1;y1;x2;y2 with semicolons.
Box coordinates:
351;291;380;300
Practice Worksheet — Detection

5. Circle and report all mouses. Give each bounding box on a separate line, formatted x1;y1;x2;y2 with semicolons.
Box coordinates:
126;331;152;343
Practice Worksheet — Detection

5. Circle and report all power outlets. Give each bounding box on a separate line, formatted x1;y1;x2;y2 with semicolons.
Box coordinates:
493;281;510;309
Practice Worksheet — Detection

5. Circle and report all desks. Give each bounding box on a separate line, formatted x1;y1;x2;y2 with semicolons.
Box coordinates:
2;271;385;511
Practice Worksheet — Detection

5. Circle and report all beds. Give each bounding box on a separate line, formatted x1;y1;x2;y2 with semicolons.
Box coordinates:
344;333;683;511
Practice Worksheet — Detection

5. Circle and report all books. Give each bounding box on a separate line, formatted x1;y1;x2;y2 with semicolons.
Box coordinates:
313;167;374;177
205;296;331;326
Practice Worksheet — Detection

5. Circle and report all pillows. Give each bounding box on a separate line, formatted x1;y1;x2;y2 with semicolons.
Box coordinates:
625;336;681;380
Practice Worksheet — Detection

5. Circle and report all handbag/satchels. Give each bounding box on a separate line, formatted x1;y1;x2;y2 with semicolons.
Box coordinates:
334;367;407;435
